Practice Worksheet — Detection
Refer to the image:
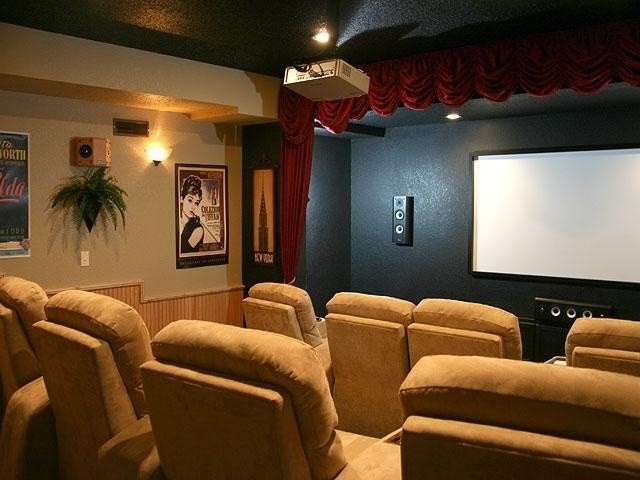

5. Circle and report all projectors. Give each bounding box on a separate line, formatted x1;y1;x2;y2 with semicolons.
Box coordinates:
283;62;371;102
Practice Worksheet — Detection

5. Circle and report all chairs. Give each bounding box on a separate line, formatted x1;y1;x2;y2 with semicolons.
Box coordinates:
326;290;415;445
241;282;333;386
407;298;524;361
336;359;638;477
139;319;381;479
1;274;50;410
565;316;638;377
2;288;161;480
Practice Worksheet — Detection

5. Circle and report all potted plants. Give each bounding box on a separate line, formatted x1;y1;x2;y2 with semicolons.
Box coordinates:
45;166;129;234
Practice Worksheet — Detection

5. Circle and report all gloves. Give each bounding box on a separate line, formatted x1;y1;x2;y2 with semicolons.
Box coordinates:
181;216;199;252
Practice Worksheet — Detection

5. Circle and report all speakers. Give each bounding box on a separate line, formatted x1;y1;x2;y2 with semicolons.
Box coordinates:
77;136;94;167
534;322;567;362
535;292;614;326
390;196;413;247
519;316;534;360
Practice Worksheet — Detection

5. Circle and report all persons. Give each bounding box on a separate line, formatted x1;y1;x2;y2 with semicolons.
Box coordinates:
210;184;217;205
180;174;205;252
